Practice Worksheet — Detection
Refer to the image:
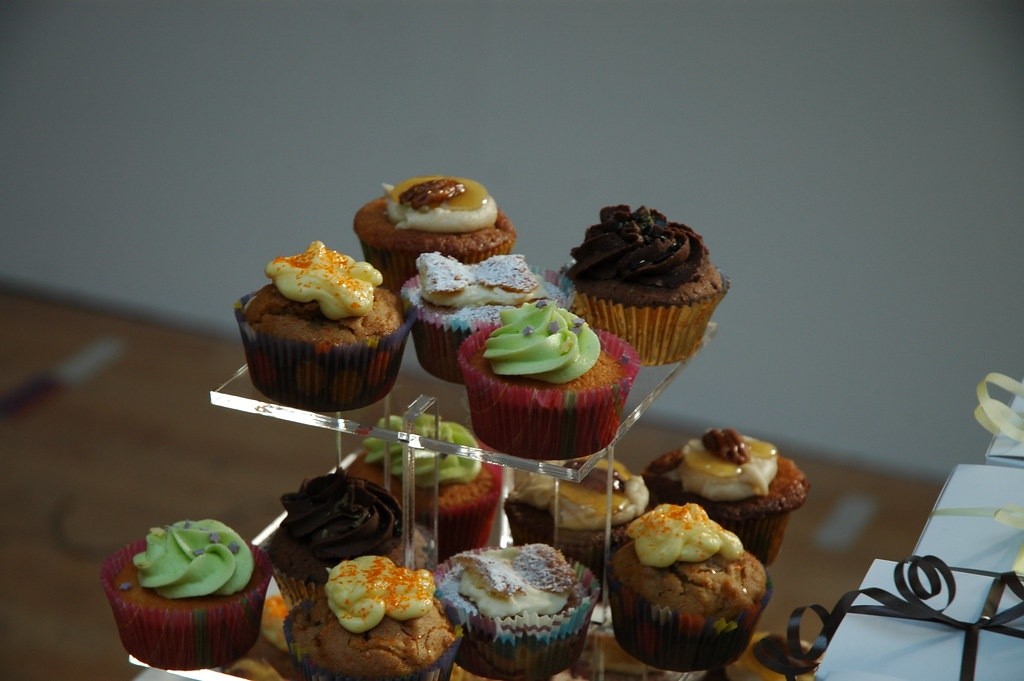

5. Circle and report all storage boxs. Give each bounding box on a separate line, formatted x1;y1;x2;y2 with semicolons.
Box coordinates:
984;375;1024;469
814;559;1024;681
905;464;1024;577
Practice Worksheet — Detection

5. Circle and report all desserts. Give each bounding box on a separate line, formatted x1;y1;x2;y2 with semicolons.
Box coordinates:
102;177;808;681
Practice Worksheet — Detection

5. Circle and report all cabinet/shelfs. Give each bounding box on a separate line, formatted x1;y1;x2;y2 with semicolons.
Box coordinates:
126;320;775;681
